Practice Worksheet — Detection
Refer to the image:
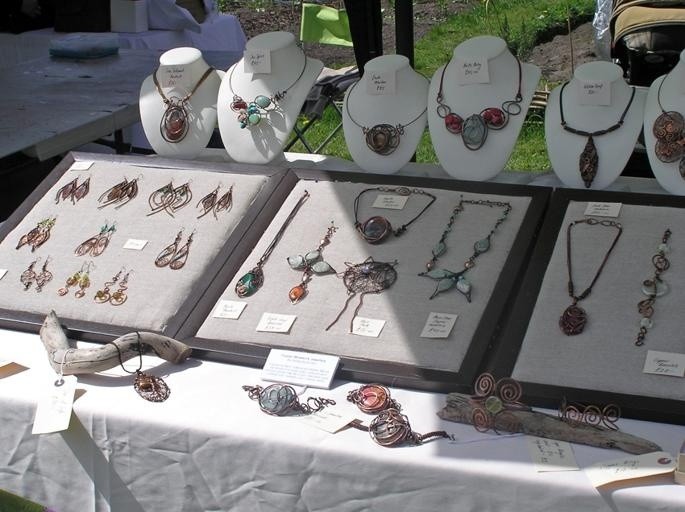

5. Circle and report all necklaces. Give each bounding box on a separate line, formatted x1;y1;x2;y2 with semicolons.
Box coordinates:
652;73;685;178
229;46;307;130
559;218;623;335
109;330;169;403
353;186;436;244
417;199;512;303
286;226;336;304
234;192;307;297
559;81;636;189
153;65;215;143
325;255;398;332
436;49;522;151
346;70;431;156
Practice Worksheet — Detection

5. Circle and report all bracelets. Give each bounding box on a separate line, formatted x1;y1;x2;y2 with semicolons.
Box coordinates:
350;407;454;447
557;395;620;430
469;372;532;435
346;384;401;412
242;384;336;416
634;228;672;345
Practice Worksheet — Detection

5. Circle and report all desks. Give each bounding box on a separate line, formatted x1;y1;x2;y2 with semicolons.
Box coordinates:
0;49;246;161
0;326;685;512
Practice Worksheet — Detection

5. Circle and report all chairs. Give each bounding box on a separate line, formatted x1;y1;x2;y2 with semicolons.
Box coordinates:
283;3;385;154
